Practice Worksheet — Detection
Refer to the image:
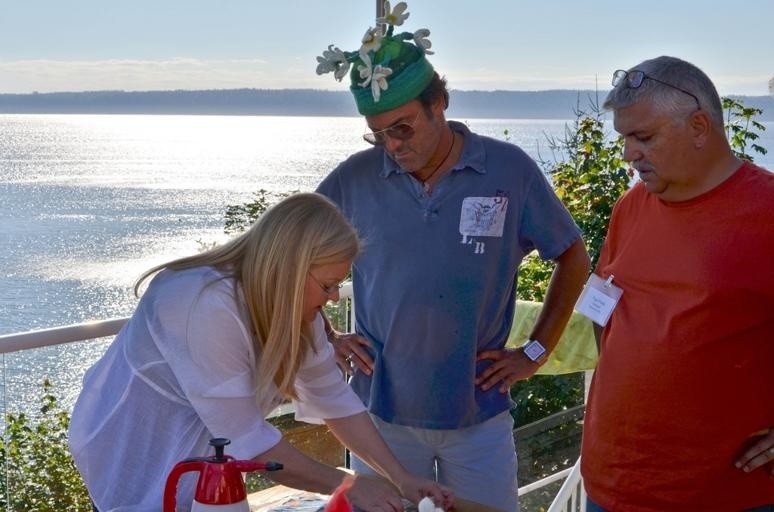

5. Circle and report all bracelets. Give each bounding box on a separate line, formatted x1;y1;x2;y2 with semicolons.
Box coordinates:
327;328;335;339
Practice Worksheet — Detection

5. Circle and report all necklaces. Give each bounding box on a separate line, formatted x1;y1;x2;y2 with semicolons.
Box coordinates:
414;130;455;192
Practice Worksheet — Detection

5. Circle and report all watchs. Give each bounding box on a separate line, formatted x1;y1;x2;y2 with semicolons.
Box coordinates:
521;338;548;368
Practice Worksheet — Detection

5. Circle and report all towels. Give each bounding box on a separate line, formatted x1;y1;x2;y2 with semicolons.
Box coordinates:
504;299;600;374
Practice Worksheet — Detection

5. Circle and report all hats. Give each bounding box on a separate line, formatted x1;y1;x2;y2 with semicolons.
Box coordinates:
316;1;435;115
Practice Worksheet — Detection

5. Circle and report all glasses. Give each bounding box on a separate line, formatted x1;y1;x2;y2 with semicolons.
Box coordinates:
308;271;347;294
612;69;701;110
363;109;424;146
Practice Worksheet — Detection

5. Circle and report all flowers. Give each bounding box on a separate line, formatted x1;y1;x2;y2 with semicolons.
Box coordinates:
315;0;435;104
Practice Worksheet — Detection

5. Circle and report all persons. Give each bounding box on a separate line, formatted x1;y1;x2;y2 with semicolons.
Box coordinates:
544;57;774;511
67;194;456;512
311;42;589;512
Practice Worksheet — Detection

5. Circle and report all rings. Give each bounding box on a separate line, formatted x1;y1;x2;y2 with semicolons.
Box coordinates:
346;352;354;361
